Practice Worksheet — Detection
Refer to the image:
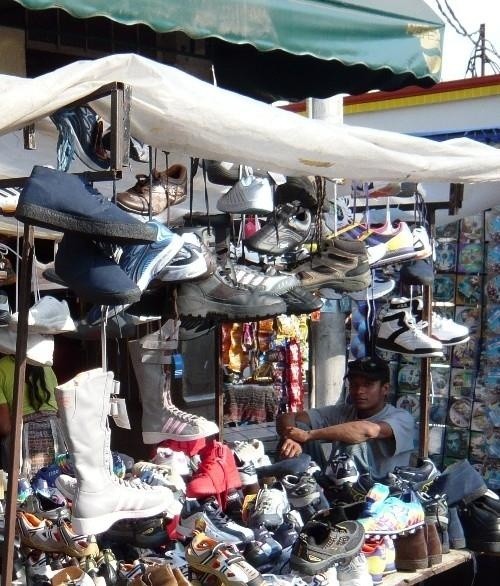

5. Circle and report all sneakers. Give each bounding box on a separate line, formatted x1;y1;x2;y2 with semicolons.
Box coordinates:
1;159;435;335
0;441;499;584
376;296;472;357
52;103;112;172
0;325;55;368
105;125;151;165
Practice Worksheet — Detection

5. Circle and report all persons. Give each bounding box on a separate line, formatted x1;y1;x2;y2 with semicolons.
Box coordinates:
0;355;68;482
276;356;414;481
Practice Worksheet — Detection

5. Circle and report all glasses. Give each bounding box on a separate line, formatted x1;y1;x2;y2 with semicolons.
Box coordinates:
348;361;381;371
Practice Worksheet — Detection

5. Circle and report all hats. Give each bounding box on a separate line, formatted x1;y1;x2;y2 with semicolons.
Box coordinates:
343;356;390;382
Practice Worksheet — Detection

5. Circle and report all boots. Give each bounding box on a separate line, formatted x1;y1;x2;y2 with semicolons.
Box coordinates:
55;367;174;538
129;317;219;444
